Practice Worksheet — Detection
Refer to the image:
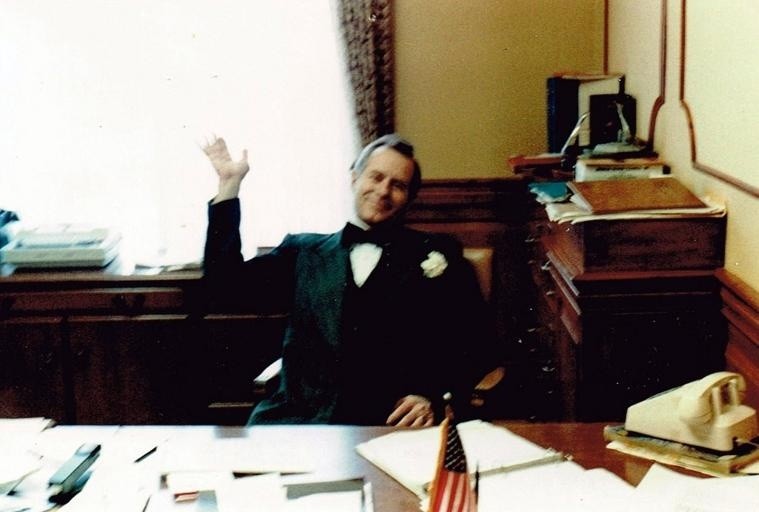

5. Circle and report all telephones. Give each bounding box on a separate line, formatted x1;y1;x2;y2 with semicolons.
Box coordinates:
624;372;758;452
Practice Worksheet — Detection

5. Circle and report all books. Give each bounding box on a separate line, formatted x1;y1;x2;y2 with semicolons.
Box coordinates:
0;227;123;267
603;425;759;475
566;175;706;215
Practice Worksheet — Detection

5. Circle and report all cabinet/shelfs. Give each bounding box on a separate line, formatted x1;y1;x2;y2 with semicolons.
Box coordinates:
509;179;727;423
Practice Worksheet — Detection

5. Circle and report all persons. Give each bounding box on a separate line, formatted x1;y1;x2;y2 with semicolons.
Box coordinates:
202;131;494;428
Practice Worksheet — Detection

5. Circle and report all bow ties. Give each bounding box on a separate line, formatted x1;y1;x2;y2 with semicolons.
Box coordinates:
341;222;385;248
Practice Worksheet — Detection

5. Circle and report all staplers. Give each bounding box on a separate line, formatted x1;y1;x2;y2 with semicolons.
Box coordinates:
47;444;101;504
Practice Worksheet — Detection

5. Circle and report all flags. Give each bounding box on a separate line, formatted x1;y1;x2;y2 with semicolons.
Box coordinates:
429;416;474;512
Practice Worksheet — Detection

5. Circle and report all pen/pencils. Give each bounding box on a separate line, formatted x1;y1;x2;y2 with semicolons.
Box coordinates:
135;447;157;463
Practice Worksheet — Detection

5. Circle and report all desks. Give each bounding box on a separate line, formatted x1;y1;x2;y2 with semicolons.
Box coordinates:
51;420;759;512
